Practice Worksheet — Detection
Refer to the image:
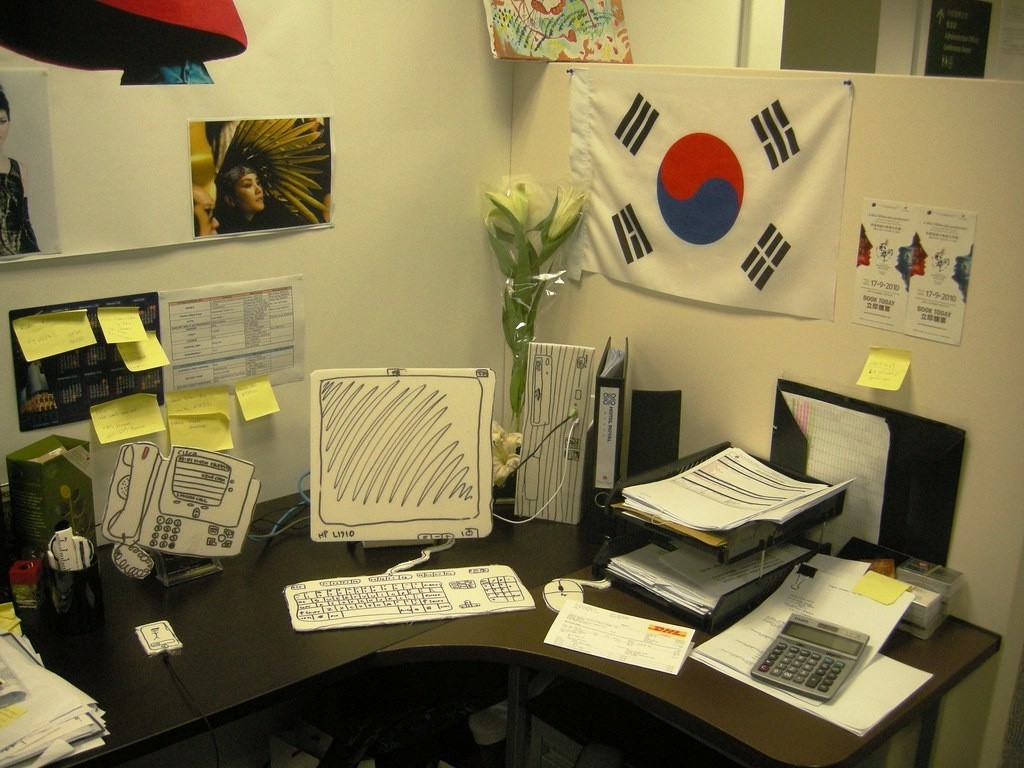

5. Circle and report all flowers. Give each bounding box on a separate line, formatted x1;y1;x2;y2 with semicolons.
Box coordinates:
483;182;585;487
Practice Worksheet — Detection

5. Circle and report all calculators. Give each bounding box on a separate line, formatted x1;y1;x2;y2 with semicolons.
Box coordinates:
750;611;870;702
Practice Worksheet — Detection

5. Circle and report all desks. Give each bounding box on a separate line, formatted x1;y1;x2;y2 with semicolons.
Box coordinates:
0;490;1002;768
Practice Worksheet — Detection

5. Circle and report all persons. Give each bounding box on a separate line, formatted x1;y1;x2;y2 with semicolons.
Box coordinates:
193;163;278;238
0;89;39;254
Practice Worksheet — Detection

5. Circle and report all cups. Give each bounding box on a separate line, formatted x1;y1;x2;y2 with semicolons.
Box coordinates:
44;555;105;632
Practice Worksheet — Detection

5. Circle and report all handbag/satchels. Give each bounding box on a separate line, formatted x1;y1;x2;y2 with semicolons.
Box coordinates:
269;717;377;767
526;714;632;768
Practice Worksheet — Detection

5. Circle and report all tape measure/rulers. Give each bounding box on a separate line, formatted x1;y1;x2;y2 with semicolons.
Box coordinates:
56;527;78;572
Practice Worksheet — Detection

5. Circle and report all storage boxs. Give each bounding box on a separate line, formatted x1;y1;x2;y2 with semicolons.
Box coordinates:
7;434;97;560
902;584;941;627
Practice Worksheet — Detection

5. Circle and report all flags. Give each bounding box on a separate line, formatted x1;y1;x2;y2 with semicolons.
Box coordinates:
564;70;855;322
